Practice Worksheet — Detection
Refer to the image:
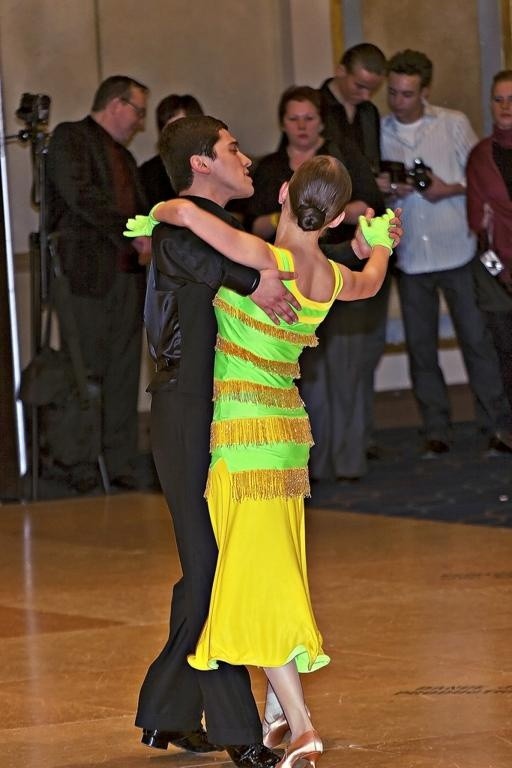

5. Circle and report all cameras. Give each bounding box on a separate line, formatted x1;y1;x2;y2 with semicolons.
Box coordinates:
409;159;433;190
379;161;404;181
15;92;50;123
480;250;505;277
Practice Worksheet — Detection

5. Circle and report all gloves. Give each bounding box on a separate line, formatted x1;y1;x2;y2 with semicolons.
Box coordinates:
356;208;399;254
123;204;164;238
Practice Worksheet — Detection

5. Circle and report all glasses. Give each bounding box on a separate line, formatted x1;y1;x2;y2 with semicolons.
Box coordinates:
119;94;148;119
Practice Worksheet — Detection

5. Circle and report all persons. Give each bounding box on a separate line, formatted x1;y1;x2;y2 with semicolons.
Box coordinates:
458;62;511;429
239;79;391;489
377;43;511;457
131;89;246;231
319;40;391;462
38;70;167;491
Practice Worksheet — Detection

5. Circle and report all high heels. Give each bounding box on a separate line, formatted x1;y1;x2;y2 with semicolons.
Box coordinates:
273;729;324;767
259;701;310;748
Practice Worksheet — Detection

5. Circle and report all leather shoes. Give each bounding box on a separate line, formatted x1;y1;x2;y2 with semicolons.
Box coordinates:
424;438;446;458
488;429;505;460
142;720;220;752
226;743;279;767
